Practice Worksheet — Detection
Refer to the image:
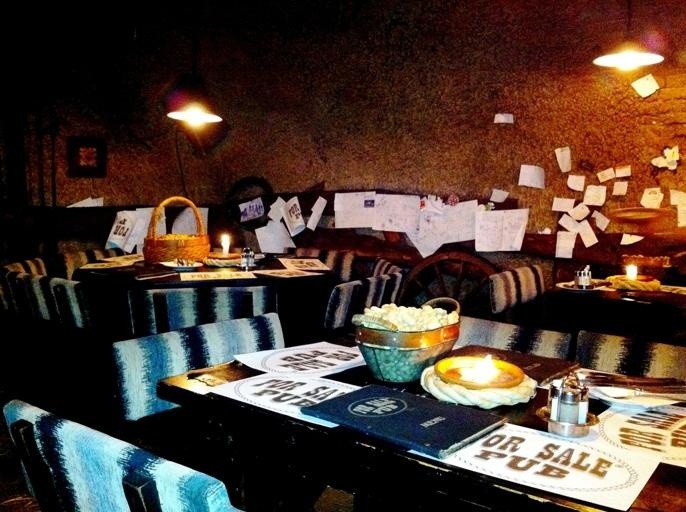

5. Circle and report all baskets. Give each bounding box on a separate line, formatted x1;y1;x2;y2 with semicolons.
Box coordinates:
143;196;211;264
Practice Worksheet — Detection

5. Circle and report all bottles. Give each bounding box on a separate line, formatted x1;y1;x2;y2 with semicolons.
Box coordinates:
240;247;254;267
548;372;588;423
574;270;594;288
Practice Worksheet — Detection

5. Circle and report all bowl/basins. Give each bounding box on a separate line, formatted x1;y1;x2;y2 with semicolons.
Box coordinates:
538;406;599;438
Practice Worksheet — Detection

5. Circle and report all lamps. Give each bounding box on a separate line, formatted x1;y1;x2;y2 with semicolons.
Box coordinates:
591;0;670;77
157;63;232;128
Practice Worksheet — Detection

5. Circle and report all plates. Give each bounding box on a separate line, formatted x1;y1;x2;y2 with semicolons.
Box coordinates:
160;261;203;271
616;276;655;282
435;355;524;390
208;252;241;260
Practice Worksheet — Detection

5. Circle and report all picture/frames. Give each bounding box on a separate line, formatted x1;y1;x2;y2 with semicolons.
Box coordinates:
63;134;110;182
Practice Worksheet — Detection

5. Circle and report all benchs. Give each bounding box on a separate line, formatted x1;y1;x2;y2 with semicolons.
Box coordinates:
3;401;242;512
451;263;684;380
111;313;286;451
1;240;405;352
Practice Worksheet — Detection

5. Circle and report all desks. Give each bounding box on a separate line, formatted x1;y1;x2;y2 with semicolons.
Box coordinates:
157;332;686;510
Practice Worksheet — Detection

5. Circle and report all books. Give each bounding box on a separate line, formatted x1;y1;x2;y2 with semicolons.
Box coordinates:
300;383;509;459
